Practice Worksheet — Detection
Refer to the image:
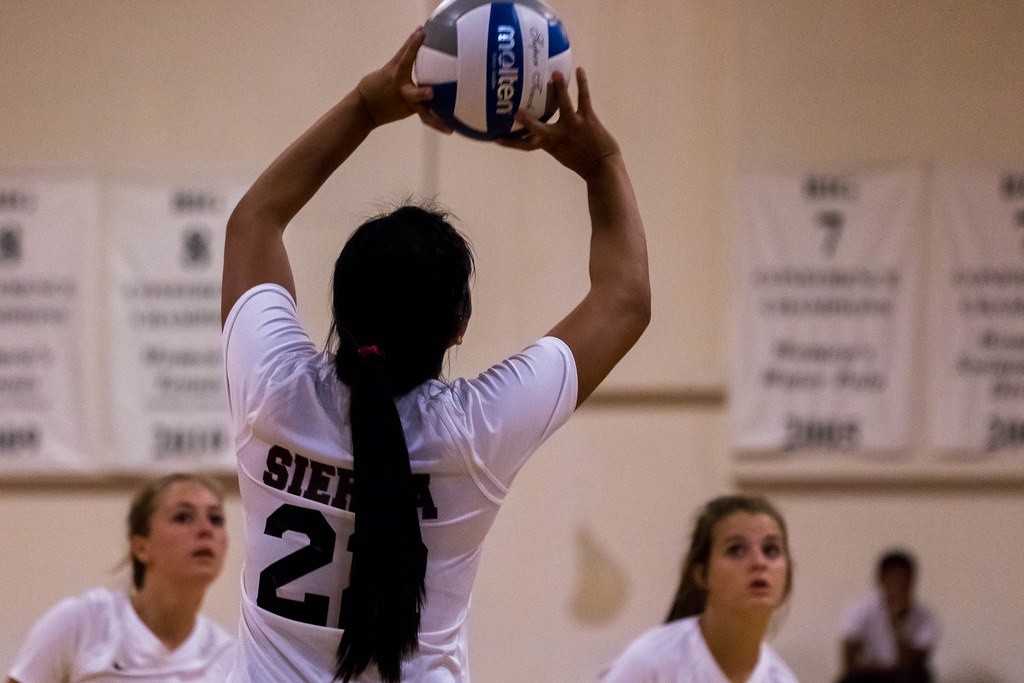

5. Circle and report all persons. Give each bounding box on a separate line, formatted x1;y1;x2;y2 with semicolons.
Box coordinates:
7;472;237;683
834;545;941;683
597;493;799;683
218;21;651;683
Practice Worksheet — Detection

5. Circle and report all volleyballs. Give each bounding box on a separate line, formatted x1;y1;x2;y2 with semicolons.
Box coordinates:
416;1;574;142
